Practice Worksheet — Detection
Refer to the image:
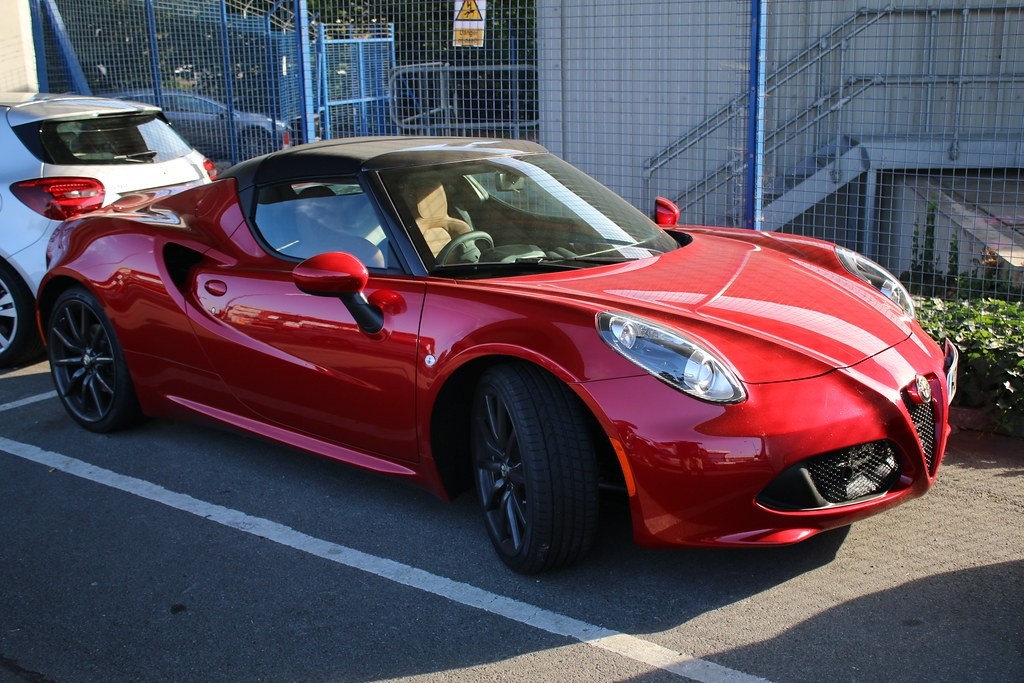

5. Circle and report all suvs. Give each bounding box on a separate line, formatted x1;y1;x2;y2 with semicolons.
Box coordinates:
0;92;216;369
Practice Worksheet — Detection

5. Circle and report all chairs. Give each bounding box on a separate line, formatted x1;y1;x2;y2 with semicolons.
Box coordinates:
389;178;475;274
295;187;383;268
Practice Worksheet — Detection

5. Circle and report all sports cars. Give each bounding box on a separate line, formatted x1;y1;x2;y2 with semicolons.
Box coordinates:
35;136;959;574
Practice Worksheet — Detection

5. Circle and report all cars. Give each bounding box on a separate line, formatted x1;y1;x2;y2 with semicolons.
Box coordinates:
291;112;320;146
91;90;288;162
